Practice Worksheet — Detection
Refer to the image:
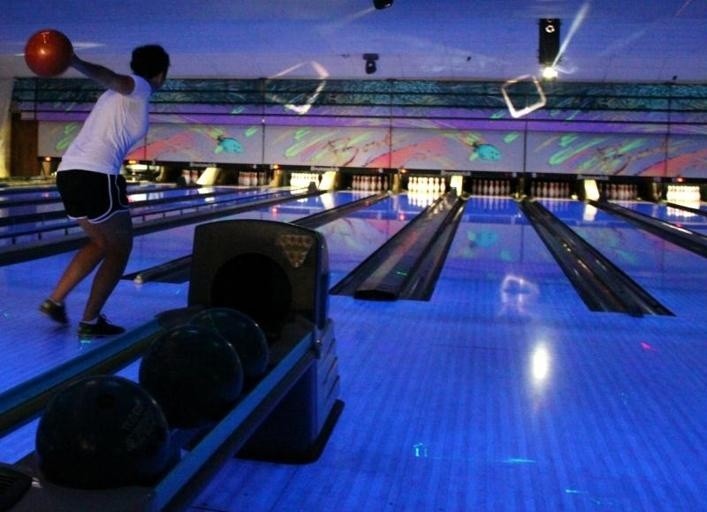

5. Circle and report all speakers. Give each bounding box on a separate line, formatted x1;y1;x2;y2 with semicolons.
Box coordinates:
539;18;560;63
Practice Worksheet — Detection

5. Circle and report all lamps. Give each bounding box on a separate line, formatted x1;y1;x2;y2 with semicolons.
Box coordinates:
372;0;394;11
362;53;378;74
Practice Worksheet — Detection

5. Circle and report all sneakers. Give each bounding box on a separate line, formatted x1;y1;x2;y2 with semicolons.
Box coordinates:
38;296;69;325
77;315;128;343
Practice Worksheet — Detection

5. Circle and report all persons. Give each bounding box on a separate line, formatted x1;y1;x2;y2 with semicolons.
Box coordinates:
38;44;170;337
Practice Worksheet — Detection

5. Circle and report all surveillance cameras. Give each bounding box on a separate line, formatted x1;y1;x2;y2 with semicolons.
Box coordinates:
366;61;375;73
